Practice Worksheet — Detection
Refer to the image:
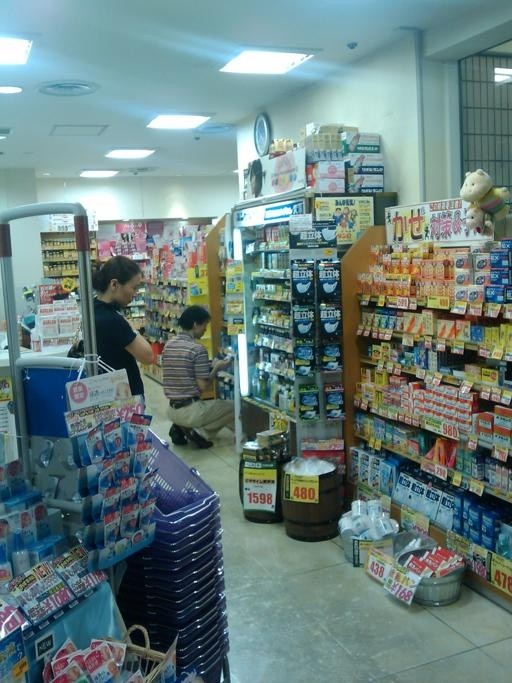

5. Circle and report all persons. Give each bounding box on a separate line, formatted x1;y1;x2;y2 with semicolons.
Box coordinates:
248;160;265;200
161;305;235;449
93;255;158;410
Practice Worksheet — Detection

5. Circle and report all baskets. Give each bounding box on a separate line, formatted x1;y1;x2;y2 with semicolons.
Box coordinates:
110;422;231;683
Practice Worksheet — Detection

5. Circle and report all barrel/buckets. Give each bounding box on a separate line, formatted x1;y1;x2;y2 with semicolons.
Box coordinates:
238;459;281;524
281;458;344;541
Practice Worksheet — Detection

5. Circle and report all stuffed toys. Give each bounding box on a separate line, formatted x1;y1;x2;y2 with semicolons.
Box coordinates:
457;166;510;219
464;207;495;236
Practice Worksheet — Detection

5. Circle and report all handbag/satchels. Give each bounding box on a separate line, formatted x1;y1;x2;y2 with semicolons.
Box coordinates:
66;336;84;358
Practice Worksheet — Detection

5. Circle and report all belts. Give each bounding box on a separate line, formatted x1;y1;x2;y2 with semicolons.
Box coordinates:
168;394;201;410
